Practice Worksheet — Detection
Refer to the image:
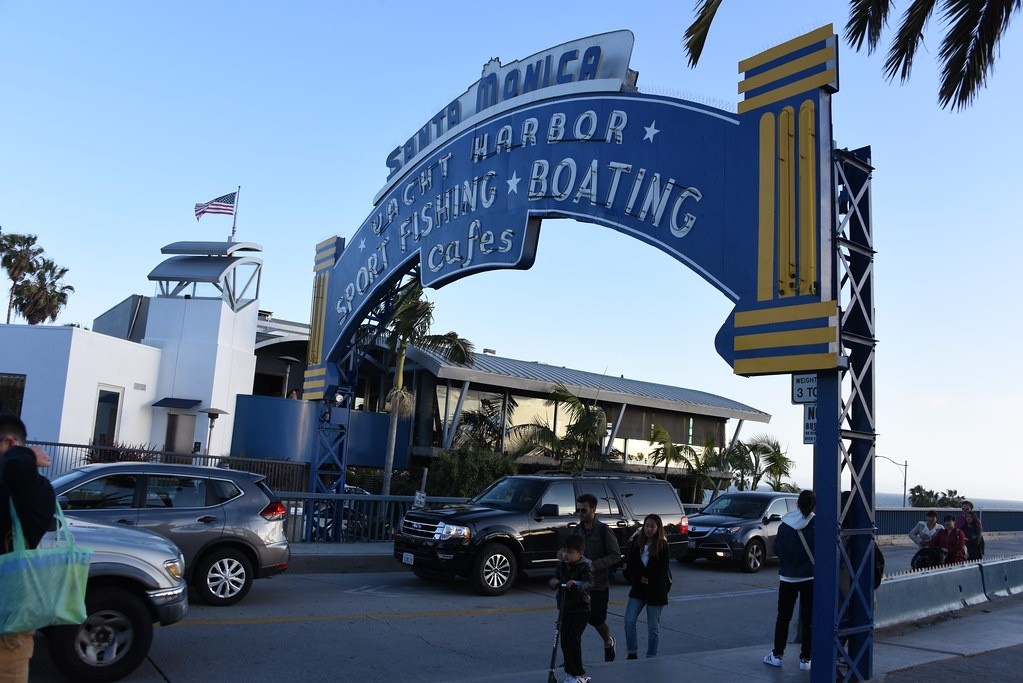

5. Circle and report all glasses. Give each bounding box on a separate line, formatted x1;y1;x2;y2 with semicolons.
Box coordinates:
961;507;971;510
577;507;593;513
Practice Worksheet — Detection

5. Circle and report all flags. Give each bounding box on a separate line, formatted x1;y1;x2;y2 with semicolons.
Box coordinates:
195;192;236;221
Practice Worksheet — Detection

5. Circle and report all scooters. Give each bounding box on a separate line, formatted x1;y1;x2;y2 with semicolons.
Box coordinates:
548;584;591;683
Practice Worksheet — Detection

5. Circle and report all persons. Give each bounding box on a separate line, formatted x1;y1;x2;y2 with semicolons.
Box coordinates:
955;501;982;562
836;490;853;667
0;414;56;683
909;510;945;551
762;490;816;671
939;515;966;566
287;389;298;399
625;514;669;660
549;533;594;683
557;493;621;662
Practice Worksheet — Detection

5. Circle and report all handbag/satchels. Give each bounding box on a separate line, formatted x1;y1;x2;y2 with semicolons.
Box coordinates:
874;542;885;589
0;493;93;634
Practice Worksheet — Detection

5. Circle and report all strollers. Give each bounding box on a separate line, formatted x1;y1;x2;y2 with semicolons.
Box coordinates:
911;547;954;573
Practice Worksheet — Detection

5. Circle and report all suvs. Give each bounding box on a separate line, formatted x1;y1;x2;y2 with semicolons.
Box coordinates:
393;469;688;596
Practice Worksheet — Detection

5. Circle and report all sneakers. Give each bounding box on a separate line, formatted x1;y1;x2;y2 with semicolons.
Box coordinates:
800;658;812;670
764;652;783;666
604;636;616;661
563;674;591;683
837;655;848;667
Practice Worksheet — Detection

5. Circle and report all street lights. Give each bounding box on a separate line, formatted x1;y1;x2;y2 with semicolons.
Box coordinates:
875;456;908;507
277;356;302;399
198;407;228;456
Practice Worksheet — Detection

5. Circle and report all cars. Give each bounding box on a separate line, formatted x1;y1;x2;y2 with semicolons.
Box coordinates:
301;505;368;541
326;486;370;509
50;462;290;607
36;514;189;683
678;491;803;573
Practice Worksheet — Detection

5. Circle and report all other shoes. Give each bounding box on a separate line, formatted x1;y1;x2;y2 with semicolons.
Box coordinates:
559;662;565;667
627;654;637;659
